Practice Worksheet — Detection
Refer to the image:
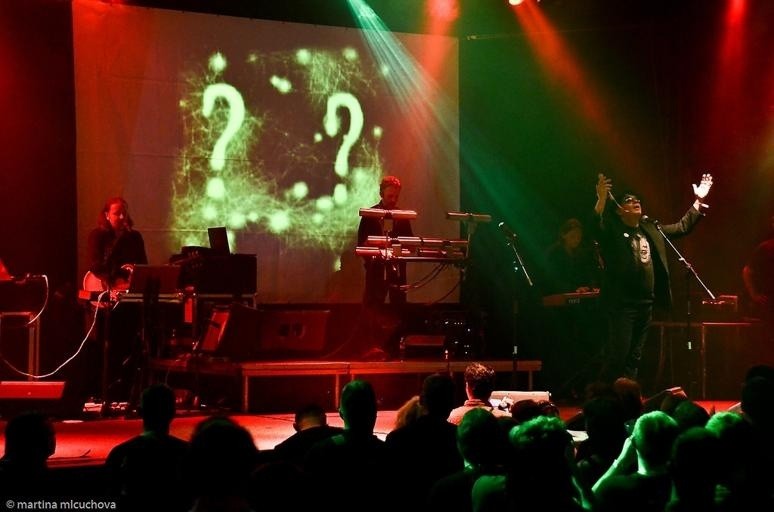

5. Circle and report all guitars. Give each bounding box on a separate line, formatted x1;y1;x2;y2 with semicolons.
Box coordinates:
83;251;204;308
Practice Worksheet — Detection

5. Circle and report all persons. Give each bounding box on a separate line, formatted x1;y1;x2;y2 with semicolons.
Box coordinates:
82;197;148;352
356;176;414;302
529;218;601;295
582;172;715;391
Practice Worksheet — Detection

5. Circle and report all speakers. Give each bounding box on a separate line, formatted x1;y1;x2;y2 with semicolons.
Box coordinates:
199;303;264;359
490;390;552;412
261;309;337;360
0;381;80;421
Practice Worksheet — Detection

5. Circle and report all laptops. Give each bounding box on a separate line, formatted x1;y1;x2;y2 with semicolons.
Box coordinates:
208;226;257;257
110;263;181;293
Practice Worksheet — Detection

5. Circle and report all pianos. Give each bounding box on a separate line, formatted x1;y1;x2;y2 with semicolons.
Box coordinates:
544;291;600;306
353;236;466;260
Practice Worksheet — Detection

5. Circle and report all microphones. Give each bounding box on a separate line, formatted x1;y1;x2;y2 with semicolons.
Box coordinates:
641;215;661;225
123;220;131;232
498;222;518;239
